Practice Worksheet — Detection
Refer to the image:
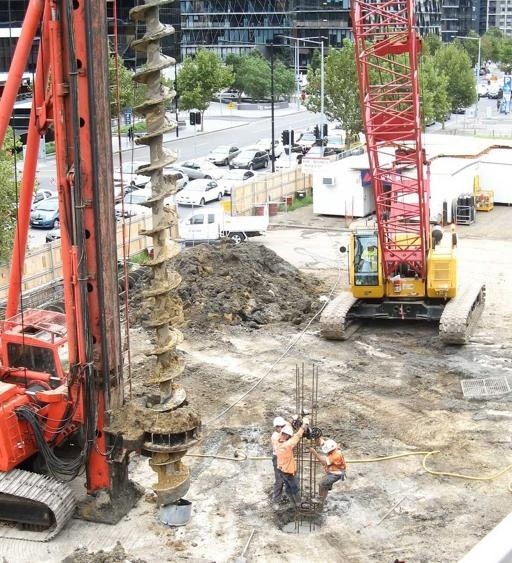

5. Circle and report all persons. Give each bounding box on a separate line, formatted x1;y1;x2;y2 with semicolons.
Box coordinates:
497;97;501;112
310;437;346;504
275;417;307;508
271;416;288;509
361;242;377;272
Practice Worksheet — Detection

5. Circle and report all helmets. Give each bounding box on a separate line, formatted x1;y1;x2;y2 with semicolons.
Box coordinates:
321;439;337;453
273;416;287;427
281;425;293;436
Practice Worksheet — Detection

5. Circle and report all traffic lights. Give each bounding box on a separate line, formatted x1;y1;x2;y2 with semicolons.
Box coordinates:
195;112;200;123
282;129;289;145
314;124;320;139
323;123;327;136
190;112;195;124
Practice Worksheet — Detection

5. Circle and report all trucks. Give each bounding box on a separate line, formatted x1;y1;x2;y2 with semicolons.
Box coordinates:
176;212;270;246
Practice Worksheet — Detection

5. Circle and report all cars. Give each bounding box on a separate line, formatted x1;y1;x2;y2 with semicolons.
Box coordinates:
30;196;59;229
476;67;503;98
11;189;53;218
113;137;283;207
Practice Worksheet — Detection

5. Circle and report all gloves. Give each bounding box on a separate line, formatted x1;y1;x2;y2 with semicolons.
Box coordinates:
302;415;309;425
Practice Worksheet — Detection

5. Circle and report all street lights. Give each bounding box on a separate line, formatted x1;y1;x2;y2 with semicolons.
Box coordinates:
450;33;482;99
276;33;324;154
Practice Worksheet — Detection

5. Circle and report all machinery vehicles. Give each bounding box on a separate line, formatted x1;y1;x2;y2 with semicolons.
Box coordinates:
319;0;487;348
0;0;203;543
